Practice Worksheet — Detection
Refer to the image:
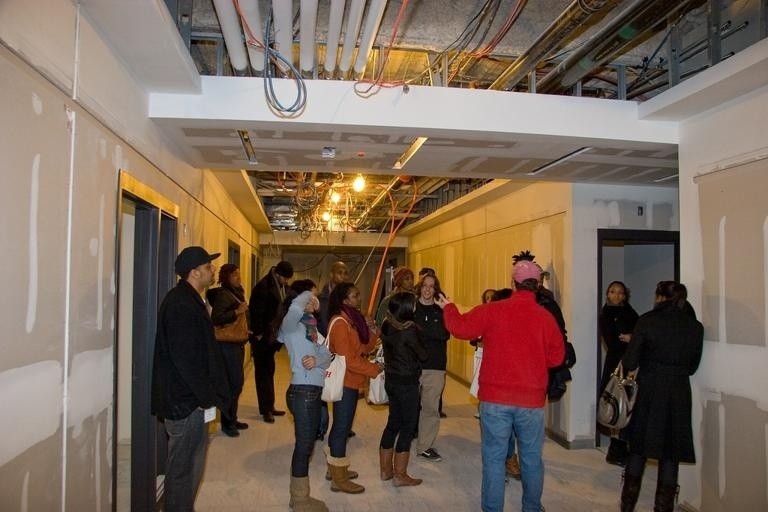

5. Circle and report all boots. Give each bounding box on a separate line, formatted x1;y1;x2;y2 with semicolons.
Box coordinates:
620;472;644;512
654;478;681;512
505;454;520;480
378;447;394;480
290;474;326;508
290;475;327;512
326;457;366;494
394;451;422;486
322;444;358;480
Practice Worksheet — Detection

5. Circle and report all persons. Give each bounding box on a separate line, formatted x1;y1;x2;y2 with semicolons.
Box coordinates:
249;261;294;424
323;283;383;494
601;281;646;466
152;246;221;512
490;288;522;485
206;265;251;438
471;289;494;419
316;261;350;338
380;291;425;488
275;279;331;512
375;268;416;338
435;260;565;511
414;267;434;293
413;272;451;462
529;263;578;403
620;279;704;512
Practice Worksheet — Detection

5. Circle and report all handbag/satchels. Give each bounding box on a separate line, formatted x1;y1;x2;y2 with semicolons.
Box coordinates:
368;345;389;404
321;317;347;402
596;359;639;430
213;290;249;343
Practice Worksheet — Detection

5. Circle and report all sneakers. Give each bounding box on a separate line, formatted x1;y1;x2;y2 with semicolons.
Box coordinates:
417;448;441;461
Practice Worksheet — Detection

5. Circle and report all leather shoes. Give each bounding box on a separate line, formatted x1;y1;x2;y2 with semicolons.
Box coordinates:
235;422;247;430
261;412;275;423
220;426;240;438
271;411;286;416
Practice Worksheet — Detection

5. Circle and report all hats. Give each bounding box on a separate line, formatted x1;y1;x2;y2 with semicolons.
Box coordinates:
175;246;221;276
511;260;541;289
